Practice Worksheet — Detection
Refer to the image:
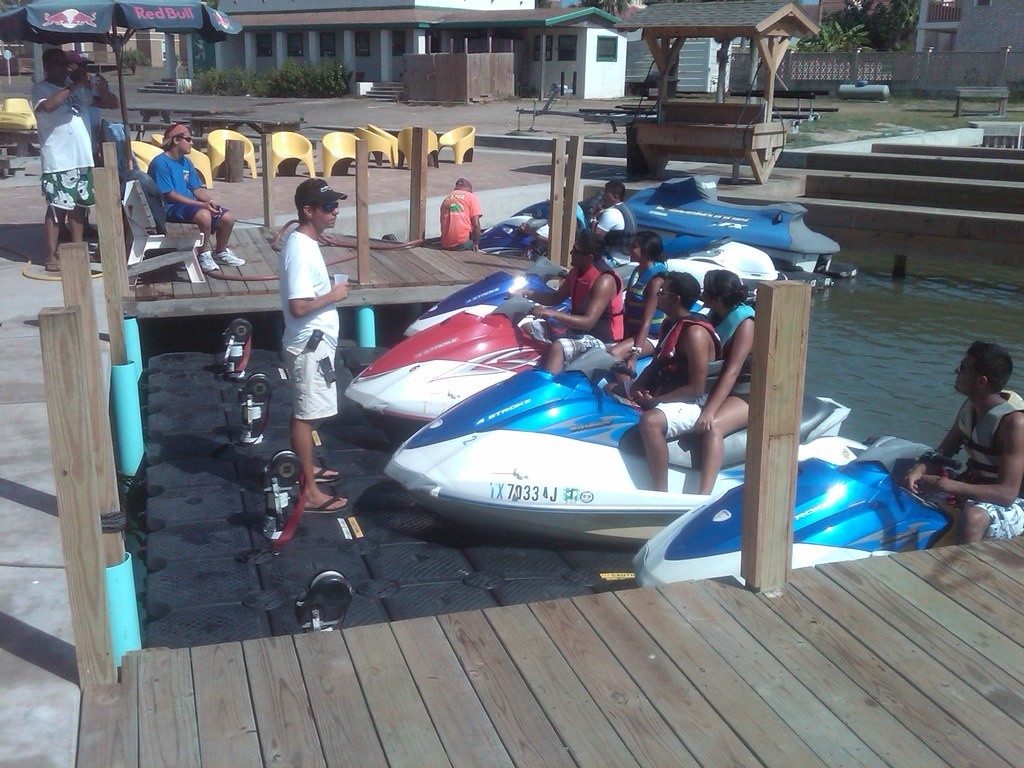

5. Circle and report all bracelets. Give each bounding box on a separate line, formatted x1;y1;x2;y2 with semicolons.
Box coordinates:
630;346;642;357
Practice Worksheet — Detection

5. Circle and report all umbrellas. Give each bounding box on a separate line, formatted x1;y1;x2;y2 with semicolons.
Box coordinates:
1;0;243;172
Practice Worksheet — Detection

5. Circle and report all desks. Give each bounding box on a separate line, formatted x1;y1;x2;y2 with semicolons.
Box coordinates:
127;103;256;142
182;116;307;176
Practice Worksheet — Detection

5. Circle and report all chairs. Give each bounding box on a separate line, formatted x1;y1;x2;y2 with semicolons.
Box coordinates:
127;124;476;190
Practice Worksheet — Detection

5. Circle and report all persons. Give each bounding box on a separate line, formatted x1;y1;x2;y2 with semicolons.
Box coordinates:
520;180;638;268
630;271;723;492
904;341;1024;549
31;47;118;271
277;178;352;513
695;269;756;495
146;124;246;272
519;230;625;377
604;231;670;373
440;178;483;252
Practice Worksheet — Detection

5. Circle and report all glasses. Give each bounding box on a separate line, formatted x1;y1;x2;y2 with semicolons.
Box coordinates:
573;244;591;256
176;135;192;143
658;285;676;296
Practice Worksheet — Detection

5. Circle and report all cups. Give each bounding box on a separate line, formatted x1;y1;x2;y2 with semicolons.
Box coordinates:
333;274;349;284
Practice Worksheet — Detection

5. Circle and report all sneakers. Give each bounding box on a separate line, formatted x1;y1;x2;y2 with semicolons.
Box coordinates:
197;251;219;273
212;247;245;266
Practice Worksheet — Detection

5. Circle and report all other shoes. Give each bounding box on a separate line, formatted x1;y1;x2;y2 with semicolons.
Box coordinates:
45;256;60;272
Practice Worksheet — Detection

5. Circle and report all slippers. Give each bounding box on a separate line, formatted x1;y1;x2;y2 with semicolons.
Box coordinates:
304;494;350;513
313;468;340;482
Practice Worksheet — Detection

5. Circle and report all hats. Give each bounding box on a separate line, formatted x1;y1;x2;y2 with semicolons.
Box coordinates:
294;178;348;206
42;48;67;63
66;50;95;64
576;230;606;258
456;178;471;187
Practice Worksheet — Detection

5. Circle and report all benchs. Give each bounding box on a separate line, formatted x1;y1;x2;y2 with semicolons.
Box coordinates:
116;122;317;176
121;178;206;286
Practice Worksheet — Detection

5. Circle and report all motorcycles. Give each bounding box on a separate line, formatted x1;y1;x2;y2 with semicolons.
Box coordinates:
632;434;961;592
471;173;839;280
344;252;837;442
384;348;853;546
